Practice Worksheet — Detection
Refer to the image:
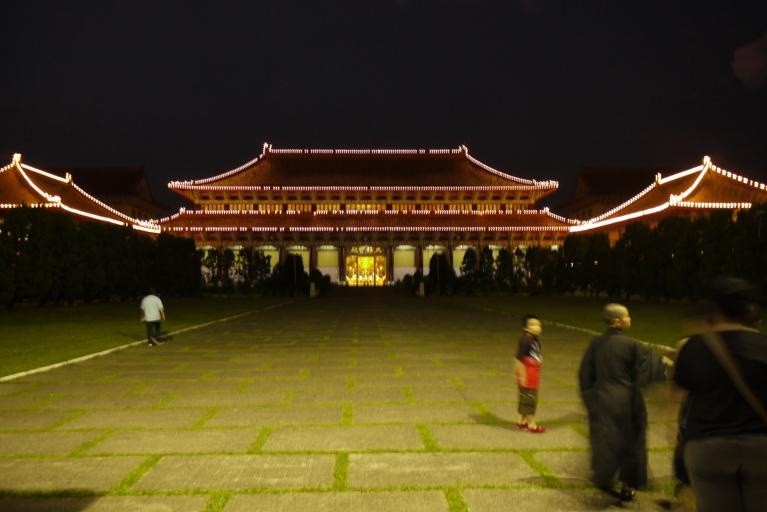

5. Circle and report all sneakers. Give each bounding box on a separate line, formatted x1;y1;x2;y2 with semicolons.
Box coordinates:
517;422;545;433
591;474;635;500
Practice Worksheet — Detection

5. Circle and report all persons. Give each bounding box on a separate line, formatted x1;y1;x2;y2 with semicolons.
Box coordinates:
510;314;546;433
666;274;765;511
137;288;166;348
579;302;652;502
